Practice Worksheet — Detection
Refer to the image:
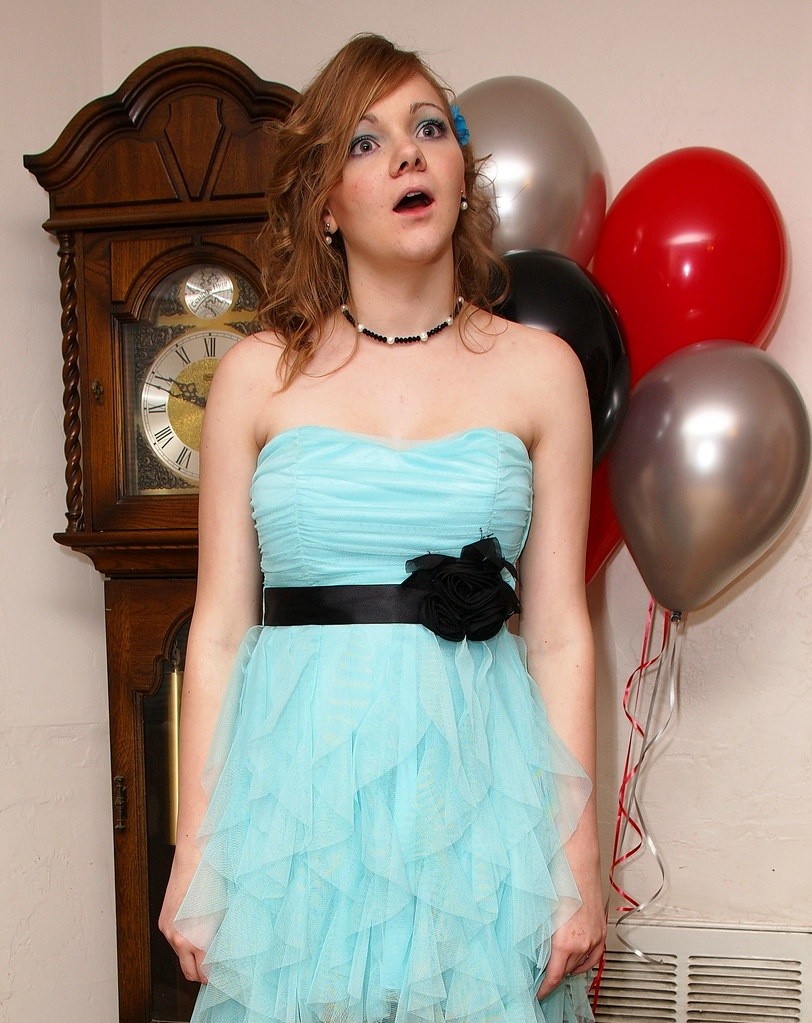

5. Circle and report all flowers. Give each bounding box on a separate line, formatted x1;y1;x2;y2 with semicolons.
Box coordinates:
397;535;522;642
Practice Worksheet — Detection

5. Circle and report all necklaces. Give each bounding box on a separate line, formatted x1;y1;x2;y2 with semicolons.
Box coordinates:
338;296;466;345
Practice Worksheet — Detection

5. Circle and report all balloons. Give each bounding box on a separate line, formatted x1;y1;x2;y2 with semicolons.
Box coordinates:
609;338;809;621
592;145;787;386
585;448;624;587
448;75;606;266
466;247;632;475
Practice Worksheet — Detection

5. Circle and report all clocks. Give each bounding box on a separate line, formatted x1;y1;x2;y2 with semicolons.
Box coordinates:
24;46;323;1023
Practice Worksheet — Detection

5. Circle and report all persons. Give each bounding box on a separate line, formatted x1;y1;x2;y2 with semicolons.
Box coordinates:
155;33;609;1023
